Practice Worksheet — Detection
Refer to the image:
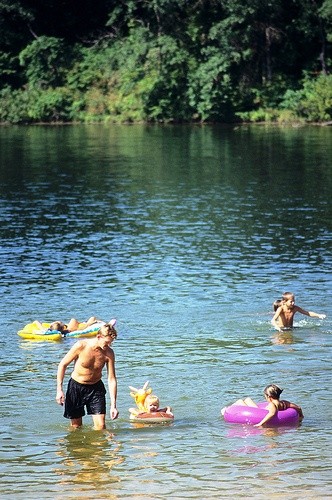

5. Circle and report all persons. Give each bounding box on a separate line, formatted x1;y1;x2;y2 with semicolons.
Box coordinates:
220;384;305;428
271;292;326;331
55;322;119;431
49;317;97;333
128;394;174;419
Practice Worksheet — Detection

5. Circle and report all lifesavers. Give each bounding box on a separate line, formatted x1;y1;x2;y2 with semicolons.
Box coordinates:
16;319;109;341
223;402;301;429
127;380;175;424
